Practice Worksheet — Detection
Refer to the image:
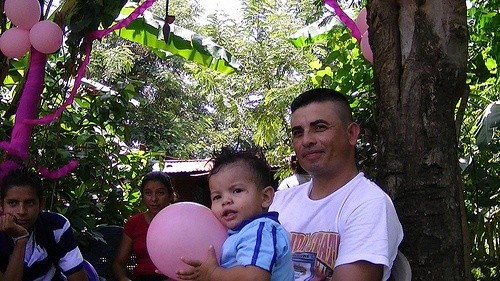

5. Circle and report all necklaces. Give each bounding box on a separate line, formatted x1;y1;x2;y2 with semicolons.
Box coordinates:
147;210;152;221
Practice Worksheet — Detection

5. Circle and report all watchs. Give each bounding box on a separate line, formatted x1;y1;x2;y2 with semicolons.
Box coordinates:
11;233;30;244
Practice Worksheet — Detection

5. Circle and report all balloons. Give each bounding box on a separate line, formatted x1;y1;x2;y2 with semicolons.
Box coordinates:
0;0;63;57
356;6;374;64
146;202;229;281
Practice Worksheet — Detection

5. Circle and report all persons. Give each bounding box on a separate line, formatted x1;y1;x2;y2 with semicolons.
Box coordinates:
270;88;405;281
112;171;183;281
0;166;91;281
176;146;295;281
272;155;311;191
